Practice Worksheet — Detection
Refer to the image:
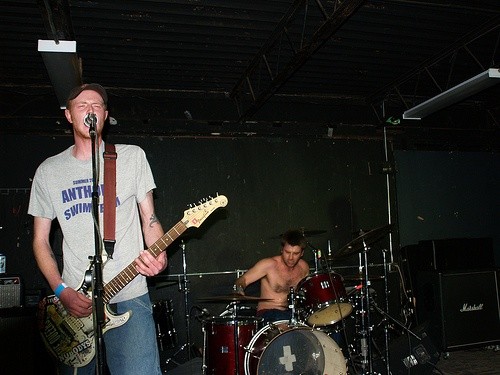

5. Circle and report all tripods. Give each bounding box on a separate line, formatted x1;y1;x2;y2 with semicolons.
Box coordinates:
164;238;204;372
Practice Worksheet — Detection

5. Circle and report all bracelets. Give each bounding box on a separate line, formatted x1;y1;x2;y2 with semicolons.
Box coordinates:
53;282;68;297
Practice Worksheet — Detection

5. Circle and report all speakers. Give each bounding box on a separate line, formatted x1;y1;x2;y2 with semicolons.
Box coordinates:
0;277;21;309
373;326;440;375
413;271;500;353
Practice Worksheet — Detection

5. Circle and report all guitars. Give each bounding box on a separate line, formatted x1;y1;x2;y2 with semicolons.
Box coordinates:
37;192;228;367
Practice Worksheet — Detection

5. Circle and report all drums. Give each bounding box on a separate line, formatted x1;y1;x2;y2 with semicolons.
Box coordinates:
244;320;347;375
295;270;353;325
204;316;265;375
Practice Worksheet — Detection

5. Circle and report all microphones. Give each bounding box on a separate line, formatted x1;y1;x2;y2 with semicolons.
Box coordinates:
84;113;98;127
196;305;211;317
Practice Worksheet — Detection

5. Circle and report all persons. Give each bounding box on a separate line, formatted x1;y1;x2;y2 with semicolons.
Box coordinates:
234;230;310;321
26;81;169;375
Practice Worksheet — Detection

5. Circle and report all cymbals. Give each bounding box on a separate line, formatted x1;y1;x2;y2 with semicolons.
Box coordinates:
199;293;274;301
344;274;385;280
273;230;327;238
335;221;398;257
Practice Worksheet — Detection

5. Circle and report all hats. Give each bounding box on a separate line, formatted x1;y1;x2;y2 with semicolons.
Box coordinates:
65;84;107;104
